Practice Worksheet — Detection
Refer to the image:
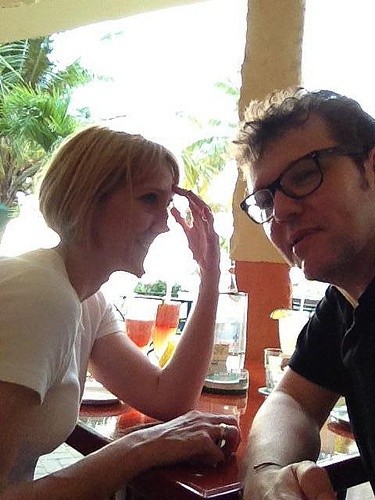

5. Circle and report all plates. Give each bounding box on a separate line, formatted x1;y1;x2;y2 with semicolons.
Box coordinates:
257;387;271;395
81;380;119;404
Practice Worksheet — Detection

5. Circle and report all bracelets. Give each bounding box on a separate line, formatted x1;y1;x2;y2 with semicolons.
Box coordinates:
238;462;285;500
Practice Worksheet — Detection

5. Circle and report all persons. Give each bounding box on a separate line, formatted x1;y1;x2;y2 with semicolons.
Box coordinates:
227;87;374;500
0;124;242;500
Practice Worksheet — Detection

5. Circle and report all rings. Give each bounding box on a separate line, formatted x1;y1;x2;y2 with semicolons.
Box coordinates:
202;216;207;222
219;422;229;439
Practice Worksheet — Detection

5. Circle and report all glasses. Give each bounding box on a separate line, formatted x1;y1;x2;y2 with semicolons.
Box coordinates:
240;144;363;224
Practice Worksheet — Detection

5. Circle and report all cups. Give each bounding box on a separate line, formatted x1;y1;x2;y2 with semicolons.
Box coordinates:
125;300;155;356
279;310;309;357
148;302;180;361
264;347;291;393
212;343;229;364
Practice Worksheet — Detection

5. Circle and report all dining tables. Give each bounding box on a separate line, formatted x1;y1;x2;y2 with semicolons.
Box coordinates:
64;359;369;500
